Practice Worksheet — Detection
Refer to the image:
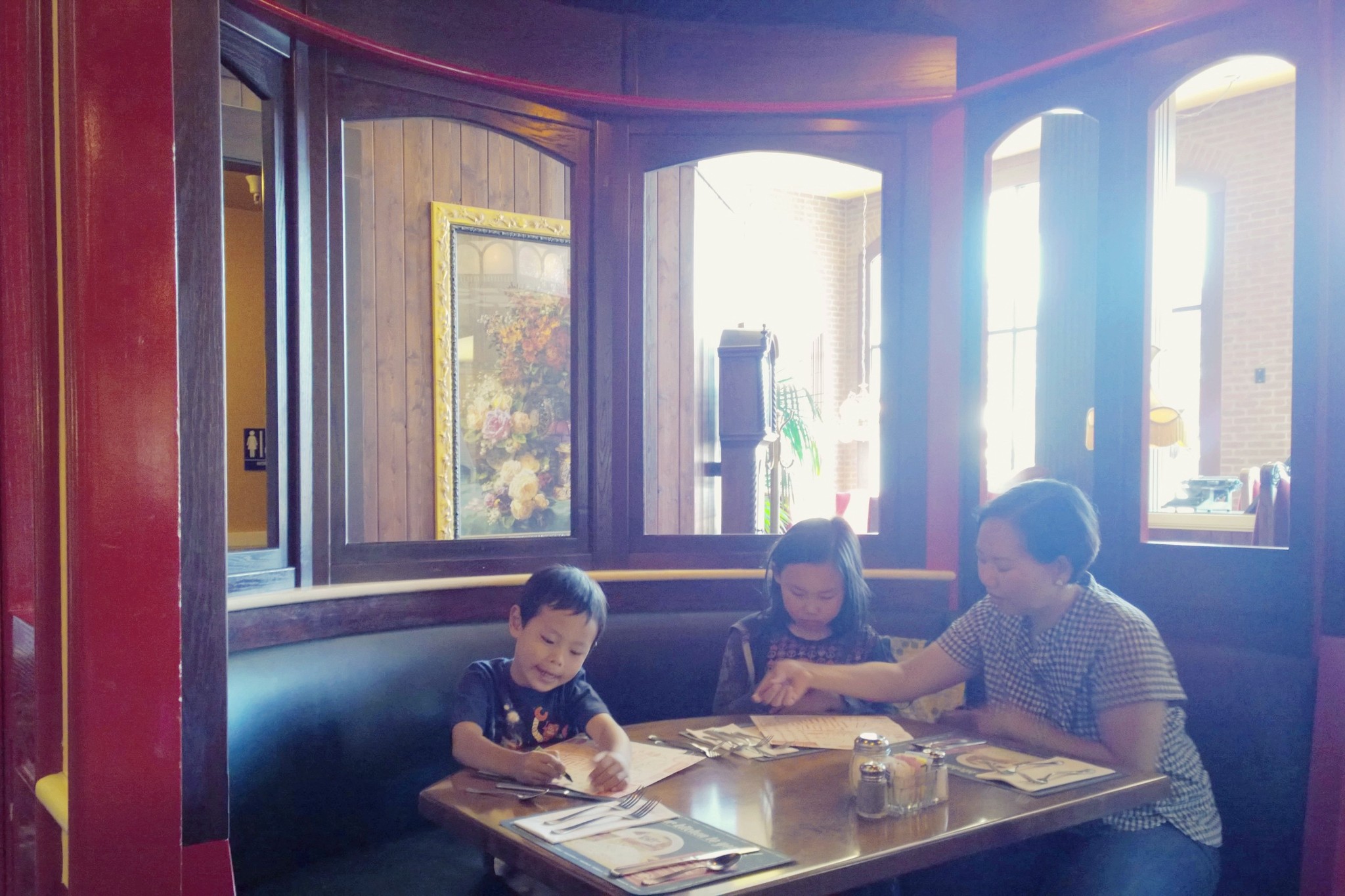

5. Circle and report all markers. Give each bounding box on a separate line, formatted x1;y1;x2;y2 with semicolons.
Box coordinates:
535;745;573;782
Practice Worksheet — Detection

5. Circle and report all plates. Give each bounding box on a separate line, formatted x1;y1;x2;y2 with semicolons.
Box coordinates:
610;827;684;854
955;753;991;770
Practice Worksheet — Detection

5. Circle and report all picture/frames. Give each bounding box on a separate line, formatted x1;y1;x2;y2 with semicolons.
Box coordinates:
429;201;571;539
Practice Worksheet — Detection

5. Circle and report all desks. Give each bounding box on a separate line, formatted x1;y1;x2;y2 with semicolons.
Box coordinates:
416;712;1172;895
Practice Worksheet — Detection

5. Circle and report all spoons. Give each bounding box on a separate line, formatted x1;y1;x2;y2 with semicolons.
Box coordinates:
642;853;741;885
910;740;968;748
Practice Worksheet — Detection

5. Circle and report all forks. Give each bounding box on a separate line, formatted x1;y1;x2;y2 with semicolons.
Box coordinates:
466;787;547;800
1019;769;1093;784
988;760;1063;774
648;729;795;758
542;785;646;825
551;797;663;834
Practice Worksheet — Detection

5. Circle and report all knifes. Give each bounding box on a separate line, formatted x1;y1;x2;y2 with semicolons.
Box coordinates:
935;741;988;750
610;847;760;878
496;782;618;801
704;730;776;757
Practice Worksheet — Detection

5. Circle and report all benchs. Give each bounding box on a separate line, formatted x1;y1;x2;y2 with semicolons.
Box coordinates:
229;610;1315;896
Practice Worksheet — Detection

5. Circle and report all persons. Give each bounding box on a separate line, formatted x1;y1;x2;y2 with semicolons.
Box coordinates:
751;479;1225;896
714;516;898;714
451;565;633;896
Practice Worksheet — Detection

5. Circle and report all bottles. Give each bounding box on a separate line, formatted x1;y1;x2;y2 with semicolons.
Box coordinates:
928;750;948;802
849;732;890;797
855;760;888;820
